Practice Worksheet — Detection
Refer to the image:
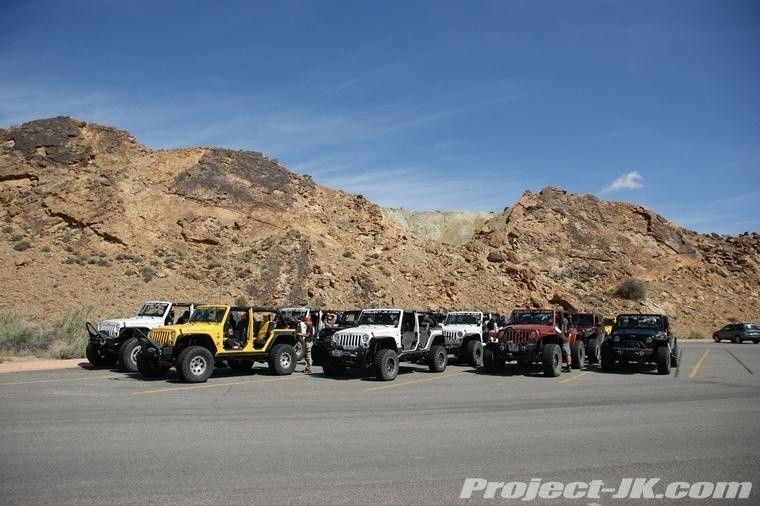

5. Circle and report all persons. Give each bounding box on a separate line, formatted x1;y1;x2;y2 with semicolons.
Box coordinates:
561;318;572;373
321;311;338;328
296;317;314;374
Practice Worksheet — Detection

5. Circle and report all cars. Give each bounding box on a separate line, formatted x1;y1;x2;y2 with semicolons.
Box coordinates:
712;323;760;343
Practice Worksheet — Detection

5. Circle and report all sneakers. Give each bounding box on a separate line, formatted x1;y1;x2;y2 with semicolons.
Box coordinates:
562;366;572;372
303;367;311;372
